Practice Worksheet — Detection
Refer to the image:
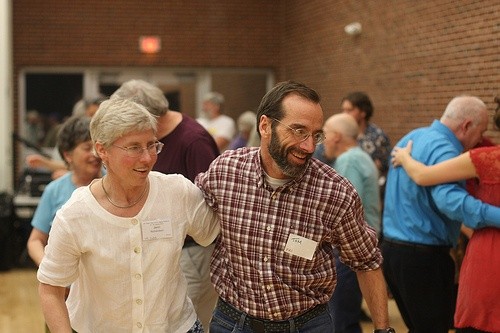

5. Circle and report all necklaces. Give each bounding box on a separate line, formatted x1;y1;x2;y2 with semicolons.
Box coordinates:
101;175;148;208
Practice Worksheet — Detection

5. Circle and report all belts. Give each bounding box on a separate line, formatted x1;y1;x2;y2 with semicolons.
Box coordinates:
217;298;330;333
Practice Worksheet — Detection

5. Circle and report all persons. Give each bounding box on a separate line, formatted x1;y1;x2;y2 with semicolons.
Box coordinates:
310;90;500;333
25;116;106;333
222;110;261;150
194;91;235;154
23;111;72;156
36;97;221;333
110;78;219;333
181;81;400;333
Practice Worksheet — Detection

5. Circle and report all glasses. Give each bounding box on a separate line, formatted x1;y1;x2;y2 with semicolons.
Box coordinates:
111;141;164;158
271;117;326;145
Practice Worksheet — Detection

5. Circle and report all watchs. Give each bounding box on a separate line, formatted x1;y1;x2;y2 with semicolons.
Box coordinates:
374;327;395;333
